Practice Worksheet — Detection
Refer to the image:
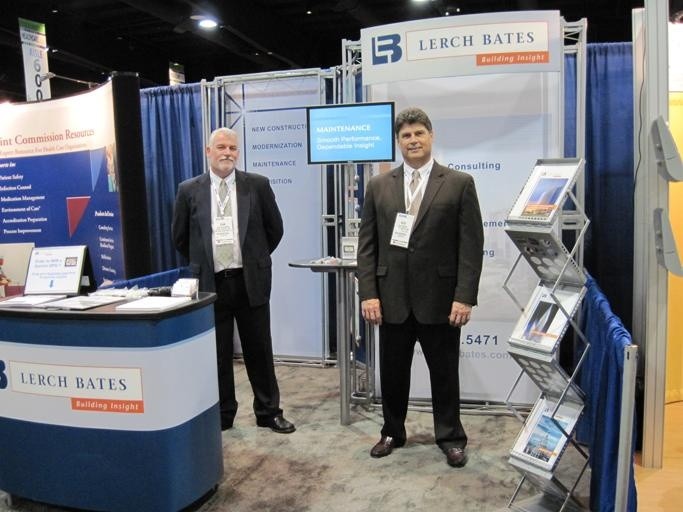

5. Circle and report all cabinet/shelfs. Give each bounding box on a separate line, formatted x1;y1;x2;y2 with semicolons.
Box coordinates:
502;156;591;512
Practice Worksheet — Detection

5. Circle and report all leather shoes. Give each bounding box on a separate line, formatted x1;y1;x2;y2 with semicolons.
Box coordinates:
446;447;466;467
370;435;405;458
271;416;295;433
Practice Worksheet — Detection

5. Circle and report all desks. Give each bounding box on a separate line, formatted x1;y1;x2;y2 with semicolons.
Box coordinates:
288;258;358;424
0;291;225;511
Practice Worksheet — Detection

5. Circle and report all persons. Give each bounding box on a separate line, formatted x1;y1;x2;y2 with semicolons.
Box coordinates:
169;127;297;436
355;106;485;468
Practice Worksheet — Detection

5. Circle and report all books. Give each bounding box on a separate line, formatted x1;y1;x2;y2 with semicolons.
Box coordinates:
510;397;583;472
115;295;192;314
0;294;66;309
508;284;578;353
507;164;578;225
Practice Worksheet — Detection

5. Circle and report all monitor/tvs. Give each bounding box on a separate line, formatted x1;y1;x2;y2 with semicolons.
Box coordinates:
304;100;395;166
67;248;98;296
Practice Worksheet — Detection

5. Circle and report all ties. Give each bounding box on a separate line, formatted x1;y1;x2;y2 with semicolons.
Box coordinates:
217;180;236;267
407;171;422;228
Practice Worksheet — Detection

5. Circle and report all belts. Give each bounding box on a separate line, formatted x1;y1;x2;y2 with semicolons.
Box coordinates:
217;269;242;279
